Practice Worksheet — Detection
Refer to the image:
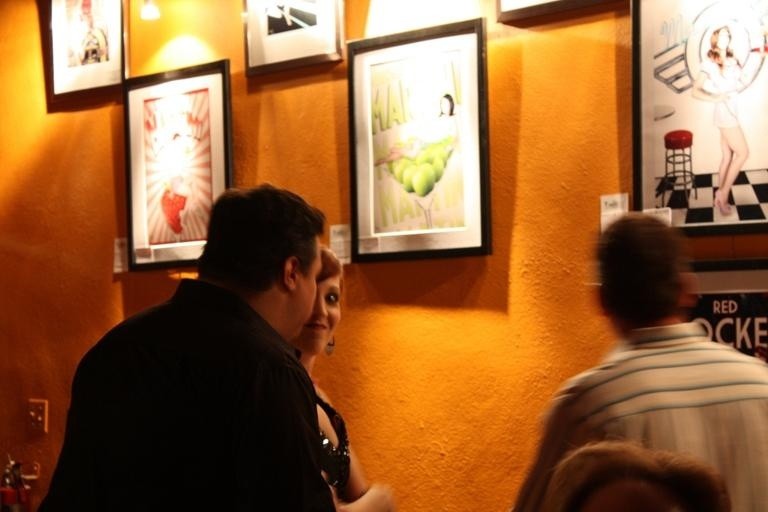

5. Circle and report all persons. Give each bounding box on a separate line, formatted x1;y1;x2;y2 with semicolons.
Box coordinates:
373;92;458;165
690;21;757;215
286;242;398;511
35;180;337;510
541;433;731;510
511;212;768;511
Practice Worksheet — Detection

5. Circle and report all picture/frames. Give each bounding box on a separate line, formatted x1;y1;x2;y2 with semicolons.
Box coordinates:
495;0;643;29
242;2;344;78
34;0;132;113
631;0;767;235
668;257;768;363
114;60;232;273
338;18;492;262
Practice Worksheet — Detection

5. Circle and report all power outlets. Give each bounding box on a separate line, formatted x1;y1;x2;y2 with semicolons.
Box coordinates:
27;399;49;433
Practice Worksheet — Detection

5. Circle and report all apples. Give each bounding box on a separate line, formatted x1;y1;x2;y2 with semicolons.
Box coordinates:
385;134;455;195
